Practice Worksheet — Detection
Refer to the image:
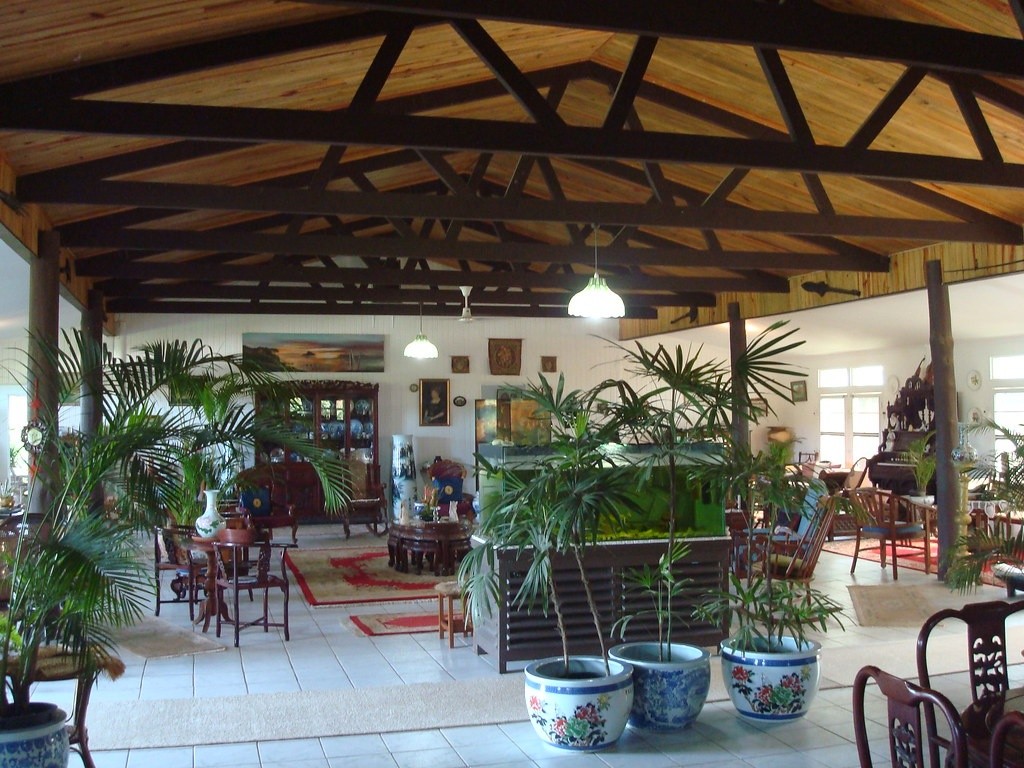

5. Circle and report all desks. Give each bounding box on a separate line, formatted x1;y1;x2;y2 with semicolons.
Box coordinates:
190;534;241;621
387;517;474;577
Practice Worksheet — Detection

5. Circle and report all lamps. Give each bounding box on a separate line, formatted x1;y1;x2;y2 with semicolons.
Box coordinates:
404;299;439;359
567;222;625;318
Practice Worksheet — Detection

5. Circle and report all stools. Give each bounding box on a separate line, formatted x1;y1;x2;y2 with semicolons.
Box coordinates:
435;582;473;649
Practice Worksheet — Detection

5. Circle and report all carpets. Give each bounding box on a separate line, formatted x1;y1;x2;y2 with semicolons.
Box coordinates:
845;583;944;628
820;532;1024;587
69;674;530;751
339;610;472;637
279;546;470;608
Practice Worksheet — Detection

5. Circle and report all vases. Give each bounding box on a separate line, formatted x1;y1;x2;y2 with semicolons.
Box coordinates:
391;433;418;522
195;490;226;538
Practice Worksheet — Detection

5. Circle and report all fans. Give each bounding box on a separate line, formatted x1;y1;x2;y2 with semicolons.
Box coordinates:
440;287;501;323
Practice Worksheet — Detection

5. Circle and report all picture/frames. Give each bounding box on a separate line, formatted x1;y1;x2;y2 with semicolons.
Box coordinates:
790;380;807;402
168;376;204;406
419;378;450;426
58;372;80;406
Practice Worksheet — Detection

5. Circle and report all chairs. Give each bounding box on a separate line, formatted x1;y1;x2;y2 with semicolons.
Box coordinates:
211;542;298;647
237;480;299;543
338;462;389;540
0;512;102;768
151;525;207;621
726;448;1024;768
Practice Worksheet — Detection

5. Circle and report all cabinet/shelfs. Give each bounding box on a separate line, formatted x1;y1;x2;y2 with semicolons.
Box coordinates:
252;381;380;524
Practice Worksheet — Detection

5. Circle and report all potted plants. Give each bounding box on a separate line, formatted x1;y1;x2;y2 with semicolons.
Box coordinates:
457;318;847;752
0;325;357;768
